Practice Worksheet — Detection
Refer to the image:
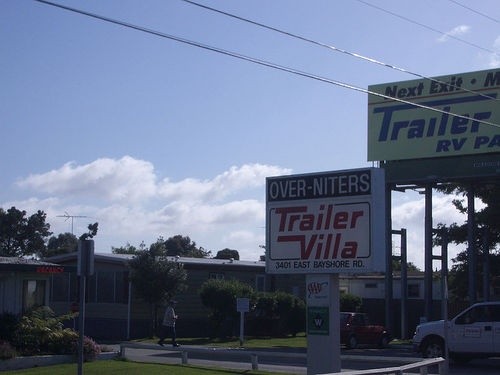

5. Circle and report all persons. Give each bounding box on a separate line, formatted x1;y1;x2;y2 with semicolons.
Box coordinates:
157;300;181;346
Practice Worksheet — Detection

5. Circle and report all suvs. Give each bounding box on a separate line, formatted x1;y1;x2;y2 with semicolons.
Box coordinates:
416;300;500;362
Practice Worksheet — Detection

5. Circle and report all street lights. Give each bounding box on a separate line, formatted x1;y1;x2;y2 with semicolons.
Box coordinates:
76;230;94;375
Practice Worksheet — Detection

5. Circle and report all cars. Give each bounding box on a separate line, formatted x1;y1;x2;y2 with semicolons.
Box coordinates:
339;310;393;349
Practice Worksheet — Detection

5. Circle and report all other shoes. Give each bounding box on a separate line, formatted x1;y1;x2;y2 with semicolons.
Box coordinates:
158;342;164;346
173;343;181;347
